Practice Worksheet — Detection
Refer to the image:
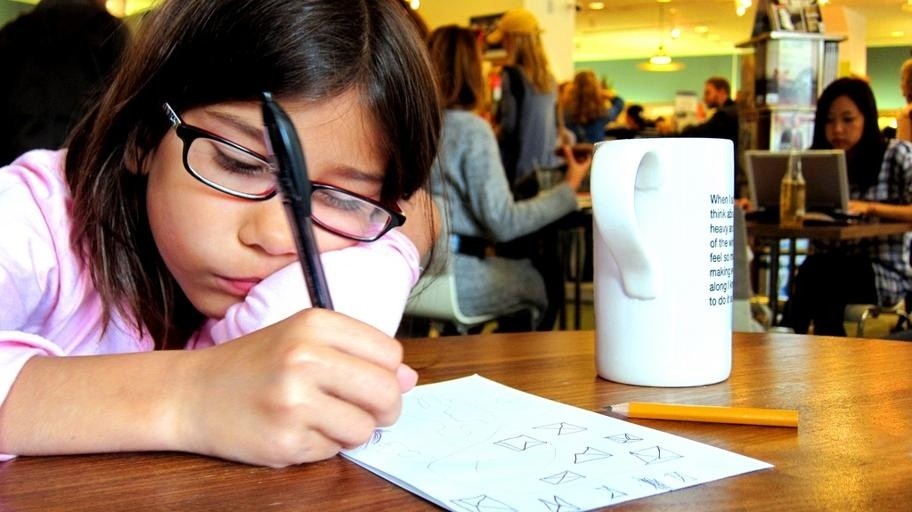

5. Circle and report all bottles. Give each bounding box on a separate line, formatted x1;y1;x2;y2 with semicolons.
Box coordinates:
779;146;806;230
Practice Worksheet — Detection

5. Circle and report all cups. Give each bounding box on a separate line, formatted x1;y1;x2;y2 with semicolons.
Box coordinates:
588;137;734;386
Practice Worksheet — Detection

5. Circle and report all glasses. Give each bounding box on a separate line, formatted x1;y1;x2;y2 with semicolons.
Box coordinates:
159;100;410;246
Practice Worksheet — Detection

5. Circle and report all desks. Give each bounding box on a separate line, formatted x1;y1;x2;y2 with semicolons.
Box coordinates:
0;332;912;512
747;224;911;327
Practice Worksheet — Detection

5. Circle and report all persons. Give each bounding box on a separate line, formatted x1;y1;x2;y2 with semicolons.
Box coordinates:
413;14;912;336
0;2;445;472
0;0;131;167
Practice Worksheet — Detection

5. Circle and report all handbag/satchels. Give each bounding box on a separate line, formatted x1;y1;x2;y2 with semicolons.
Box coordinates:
494;126;525;192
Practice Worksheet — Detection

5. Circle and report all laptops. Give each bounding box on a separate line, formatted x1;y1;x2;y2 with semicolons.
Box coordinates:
744;148;856;220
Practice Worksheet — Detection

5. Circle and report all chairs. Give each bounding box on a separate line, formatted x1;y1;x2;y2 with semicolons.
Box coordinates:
404;196;536;336
843;297;907;337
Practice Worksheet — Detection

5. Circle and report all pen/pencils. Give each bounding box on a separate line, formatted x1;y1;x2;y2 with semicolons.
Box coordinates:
261;92;336;311
602;401;800;427
